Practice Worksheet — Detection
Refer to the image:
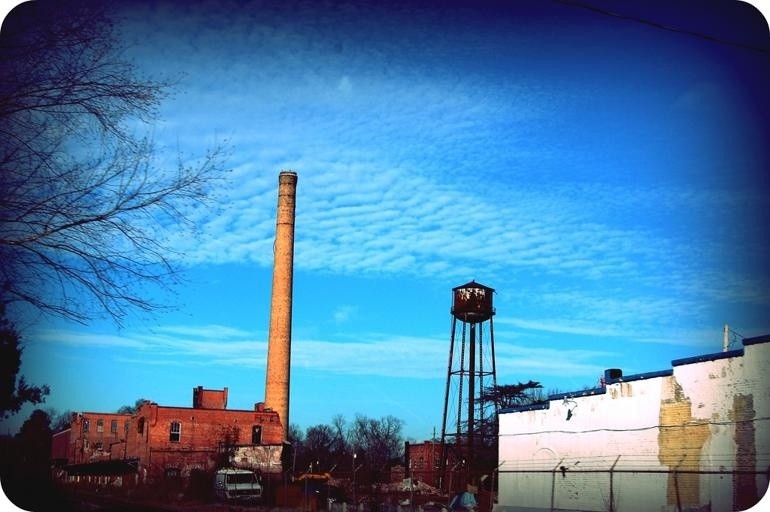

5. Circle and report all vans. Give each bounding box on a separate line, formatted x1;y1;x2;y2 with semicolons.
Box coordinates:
212;467;264;503
